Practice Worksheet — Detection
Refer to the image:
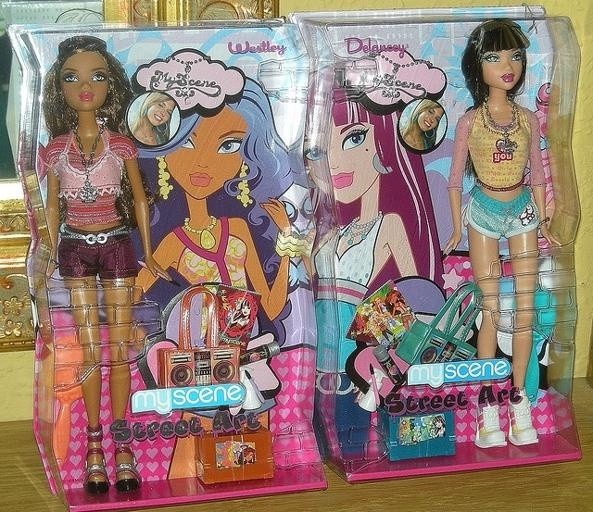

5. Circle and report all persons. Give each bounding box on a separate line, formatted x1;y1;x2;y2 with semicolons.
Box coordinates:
131;90;176;144
401;100;445;150
41;36;171;491
443;19;561;449
